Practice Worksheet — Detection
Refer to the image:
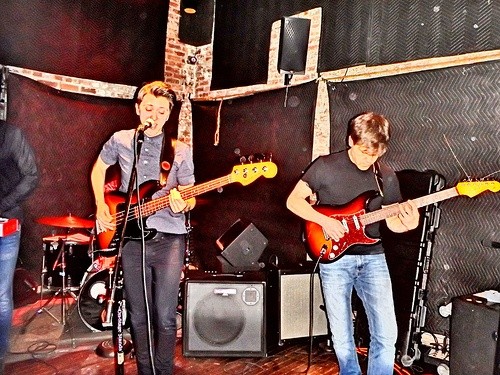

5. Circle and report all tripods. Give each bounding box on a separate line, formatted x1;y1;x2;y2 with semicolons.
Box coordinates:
19;228;78;334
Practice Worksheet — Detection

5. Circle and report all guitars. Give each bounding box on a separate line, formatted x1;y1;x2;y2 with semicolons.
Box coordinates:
79;257;130;332
302;178;500;264
96;152;279;251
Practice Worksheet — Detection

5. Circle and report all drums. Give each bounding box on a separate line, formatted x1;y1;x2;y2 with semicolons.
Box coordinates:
41;235;96;292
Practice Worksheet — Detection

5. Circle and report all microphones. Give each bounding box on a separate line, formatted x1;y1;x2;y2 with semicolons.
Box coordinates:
138;119;155;131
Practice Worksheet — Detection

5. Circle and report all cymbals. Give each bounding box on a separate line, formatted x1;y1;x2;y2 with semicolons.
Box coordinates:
35;216;96;229
185;220;201;226
196;197;211;206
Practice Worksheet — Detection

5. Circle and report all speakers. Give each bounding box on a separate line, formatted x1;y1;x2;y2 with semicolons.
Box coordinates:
278;270;330;346
178;0;214;47
437;291;500;375
216;219;268;267
277;17;311;75
182;279;267;357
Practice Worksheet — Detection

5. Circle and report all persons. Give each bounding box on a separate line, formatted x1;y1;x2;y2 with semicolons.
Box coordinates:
286;112;420;375
0;118;38;366
90;81;195;375
